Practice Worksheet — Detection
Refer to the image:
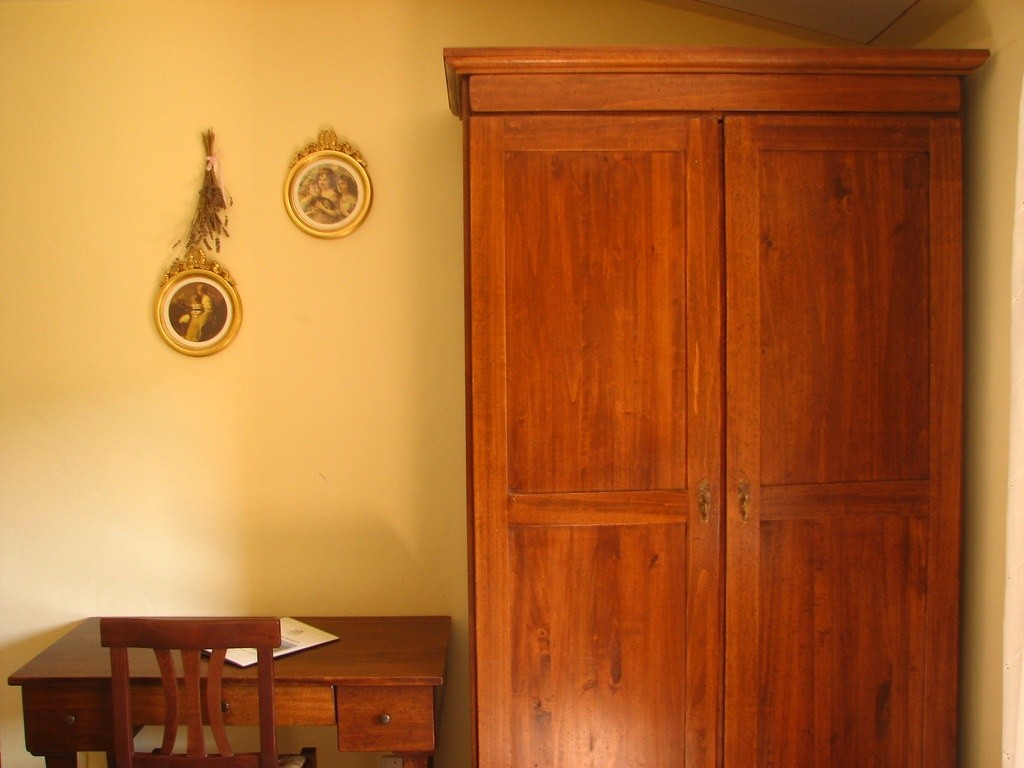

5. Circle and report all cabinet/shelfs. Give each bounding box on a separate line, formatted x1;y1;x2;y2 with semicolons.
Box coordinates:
443;47;992;768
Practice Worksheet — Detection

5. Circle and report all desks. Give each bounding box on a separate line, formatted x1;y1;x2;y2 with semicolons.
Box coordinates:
7;616;450;768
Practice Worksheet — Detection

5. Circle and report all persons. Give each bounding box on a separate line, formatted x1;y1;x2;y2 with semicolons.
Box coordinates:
299;167;357;225
178;283;215;344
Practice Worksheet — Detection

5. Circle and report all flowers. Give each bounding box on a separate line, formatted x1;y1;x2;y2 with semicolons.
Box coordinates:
171;127;234;260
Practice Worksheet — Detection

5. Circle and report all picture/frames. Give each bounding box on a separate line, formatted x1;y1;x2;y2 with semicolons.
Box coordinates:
282;128;374;240
154;248;242;357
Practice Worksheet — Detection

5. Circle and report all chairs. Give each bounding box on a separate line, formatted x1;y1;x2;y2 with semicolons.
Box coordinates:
99;616;318;768
